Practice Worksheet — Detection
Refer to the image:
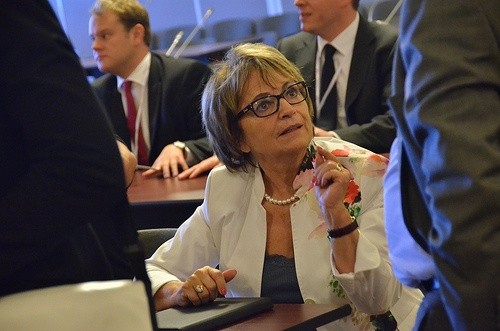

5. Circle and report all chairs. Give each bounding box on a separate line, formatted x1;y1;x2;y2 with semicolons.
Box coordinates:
133;228;178;259
158;0;404;49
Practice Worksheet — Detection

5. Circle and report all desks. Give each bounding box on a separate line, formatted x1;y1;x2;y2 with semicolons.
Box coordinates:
79;38;264;76
127;168;208;231
159;296;351;331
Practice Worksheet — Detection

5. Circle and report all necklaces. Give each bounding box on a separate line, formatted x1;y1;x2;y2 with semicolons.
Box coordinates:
262;192;299;205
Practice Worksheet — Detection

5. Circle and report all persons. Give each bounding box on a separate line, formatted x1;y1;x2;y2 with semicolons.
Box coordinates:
176;0;400;181
0;0;159;330
382;0;500;331
144;41;425;331
88;0;215;179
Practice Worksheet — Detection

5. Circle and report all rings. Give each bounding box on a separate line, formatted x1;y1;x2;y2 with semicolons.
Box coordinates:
336;162;344;171
194;284;205;293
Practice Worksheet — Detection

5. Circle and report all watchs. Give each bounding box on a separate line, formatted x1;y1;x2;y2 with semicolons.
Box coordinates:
173;139;187;159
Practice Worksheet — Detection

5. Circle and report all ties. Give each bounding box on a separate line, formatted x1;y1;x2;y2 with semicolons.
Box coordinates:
318;43;339;131
121;81;149;165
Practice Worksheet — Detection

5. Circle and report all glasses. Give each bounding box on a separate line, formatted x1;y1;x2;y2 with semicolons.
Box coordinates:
230;80;311;123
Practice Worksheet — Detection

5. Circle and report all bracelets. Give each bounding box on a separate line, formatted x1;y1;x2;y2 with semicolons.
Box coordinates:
327;215;359;239
